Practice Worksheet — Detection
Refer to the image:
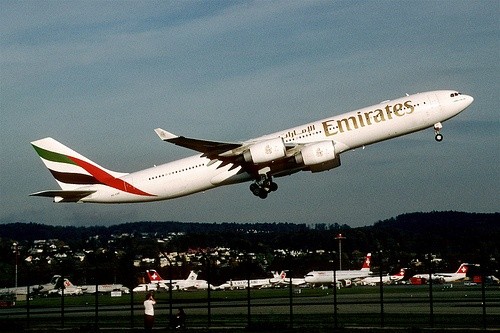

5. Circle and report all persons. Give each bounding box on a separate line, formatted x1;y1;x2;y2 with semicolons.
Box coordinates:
168;306;185;329
143;292;156;333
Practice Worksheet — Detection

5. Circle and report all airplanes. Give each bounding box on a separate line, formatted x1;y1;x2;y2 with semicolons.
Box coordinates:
27;88;476;206
0;252;498;299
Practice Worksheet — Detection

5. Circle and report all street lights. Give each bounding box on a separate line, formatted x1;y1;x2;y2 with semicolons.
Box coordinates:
12;241;19;287
334;234;346;270
